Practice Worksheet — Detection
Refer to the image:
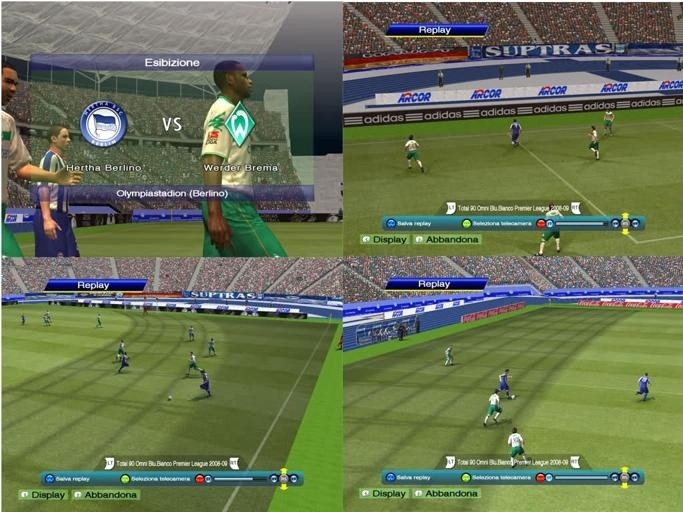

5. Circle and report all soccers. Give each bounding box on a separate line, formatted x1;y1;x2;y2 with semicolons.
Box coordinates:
511;395;516;399
168;395;172;400
